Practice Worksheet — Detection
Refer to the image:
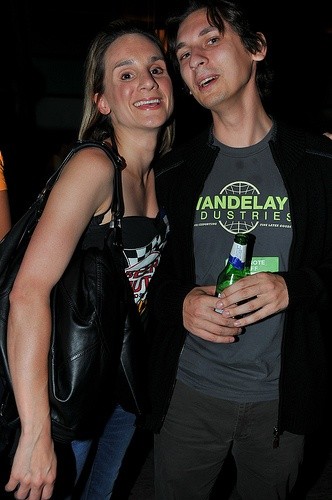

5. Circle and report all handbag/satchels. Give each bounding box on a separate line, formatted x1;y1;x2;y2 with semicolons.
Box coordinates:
0;142;129;441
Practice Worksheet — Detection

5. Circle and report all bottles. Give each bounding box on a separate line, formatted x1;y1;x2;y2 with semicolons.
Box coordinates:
214;233;250;320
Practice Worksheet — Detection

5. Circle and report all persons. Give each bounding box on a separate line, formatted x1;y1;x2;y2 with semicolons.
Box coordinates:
134;0;332;500
1;26;332;500
